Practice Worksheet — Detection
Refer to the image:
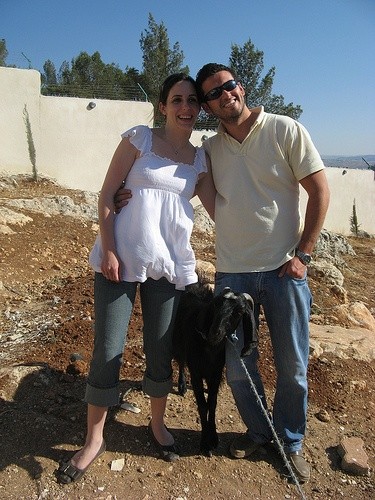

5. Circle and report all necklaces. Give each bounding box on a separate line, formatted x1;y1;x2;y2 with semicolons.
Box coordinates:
175;142;186;154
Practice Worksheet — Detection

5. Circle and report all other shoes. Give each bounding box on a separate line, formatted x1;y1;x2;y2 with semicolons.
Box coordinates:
58;437;107;483
147;419;178;462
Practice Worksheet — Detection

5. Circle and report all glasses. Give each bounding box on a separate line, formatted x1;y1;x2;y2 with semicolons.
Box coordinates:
203;79;238;102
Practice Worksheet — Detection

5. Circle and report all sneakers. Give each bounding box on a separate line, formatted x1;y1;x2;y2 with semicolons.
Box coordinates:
230;433;258;459
288;452;310;482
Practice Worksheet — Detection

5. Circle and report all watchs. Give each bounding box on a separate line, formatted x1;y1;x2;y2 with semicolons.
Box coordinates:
296;250;311;265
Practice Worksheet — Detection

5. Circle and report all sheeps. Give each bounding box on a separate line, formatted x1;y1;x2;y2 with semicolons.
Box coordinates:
169;276;258;453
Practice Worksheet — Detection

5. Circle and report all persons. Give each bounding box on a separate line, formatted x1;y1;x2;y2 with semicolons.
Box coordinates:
114;63;329;482
58;73;217;484
